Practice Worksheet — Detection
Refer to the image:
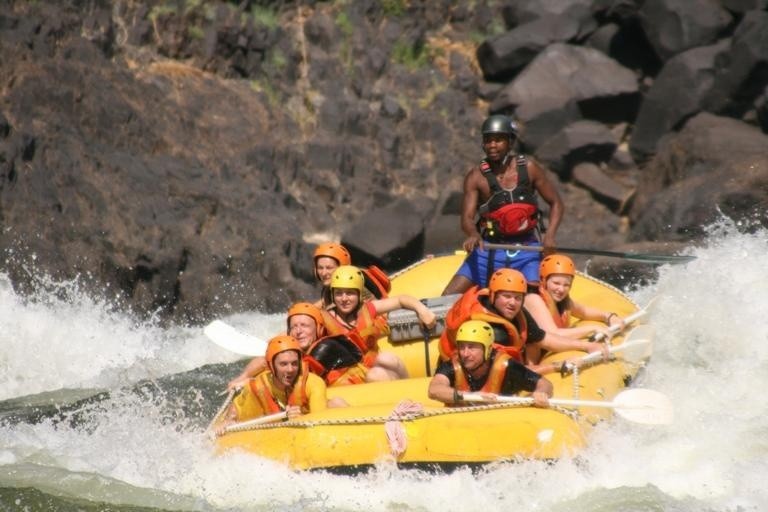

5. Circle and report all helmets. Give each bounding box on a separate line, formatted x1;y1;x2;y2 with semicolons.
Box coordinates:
265;335;303;376
330;266;364;304
488;268;528;308
480;116;518;145
285;301;324;339
454;319;495;363
312;241;350;279
540;255;577;290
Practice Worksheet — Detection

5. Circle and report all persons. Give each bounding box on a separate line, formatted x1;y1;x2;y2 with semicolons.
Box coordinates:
312;242;376;311
227;303;363;384
429;319;553;408
524;255;625;339
316;264;436;382
225;335;346;428
437;267;612;374
441;114;565;297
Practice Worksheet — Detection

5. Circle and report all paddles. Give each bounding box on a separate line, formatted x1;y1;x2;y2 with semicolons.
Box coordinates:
563;325;654;375
463;388;672;426
485;244;696;266
588;297;657;345
